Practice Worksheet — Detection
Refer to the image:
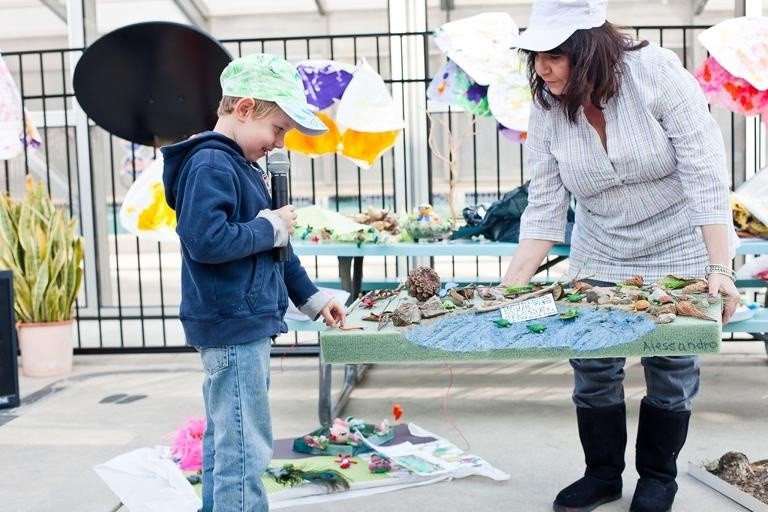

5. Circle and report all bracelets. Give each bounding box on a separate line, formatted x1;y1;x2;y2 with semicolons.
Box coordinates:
702;264;736;279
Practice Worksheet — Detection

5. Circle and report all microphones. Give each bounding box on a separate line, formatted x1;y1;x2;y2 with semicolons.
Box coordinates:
268;153;290;262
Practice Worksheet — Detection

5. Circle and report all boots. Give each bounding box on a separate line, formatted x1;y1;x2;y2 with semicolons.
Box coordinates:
547;404;631;512
626;397;695;512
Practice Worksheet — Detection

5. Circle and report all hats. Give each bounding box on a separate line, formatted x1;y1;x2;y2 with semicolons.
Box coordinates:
219;53;330;137
512;0;608;52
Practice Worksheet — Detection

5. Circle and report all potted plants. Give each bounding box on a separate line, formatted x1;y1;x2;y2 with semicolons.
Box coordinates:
1;173;85;378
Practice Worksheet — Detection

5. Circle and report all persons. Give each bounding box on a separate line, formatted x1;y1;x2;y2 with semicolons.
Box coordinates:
159;53;346;512
500;1;740;512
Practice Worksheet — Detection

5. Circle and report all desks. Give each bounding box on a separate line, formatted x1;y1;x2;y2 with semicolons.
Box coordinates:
290;223;768;387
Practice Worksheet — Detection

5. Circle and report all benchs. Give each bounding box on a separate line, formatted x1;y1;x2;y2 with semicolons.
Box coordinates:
309;276;768;387
284;299;768;429
